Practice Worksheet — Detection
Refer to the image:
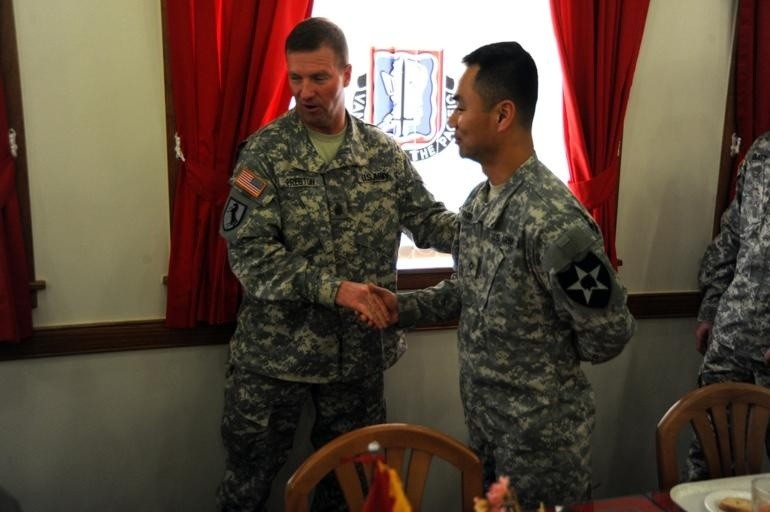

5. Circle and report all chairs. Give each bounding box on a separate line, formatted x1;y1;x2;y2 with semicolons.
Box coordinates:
287;422;487;512
651;381;769;488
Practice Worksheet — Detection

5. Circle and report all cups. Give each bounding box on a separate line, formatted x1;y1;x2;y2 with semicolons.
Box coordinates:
752;477;770;512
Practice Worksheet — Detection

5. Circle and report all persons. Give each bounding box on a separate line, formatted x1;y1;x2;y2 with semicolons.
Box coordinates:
352;39;640;512
683;128;769;479
213;15;458;510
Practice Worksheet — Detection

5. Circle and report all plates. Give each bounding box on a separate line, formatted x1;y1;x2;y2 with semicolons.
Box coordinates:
704;488;754;512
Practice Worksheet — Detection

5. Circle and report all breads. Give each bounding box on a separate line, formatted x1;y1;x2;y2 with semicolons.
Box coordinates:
718;496;752;512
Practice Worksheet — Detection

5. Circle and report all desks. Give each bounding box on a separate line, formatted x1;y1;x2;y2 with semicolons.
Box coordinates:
556;490;661;512
646;473;768;511
